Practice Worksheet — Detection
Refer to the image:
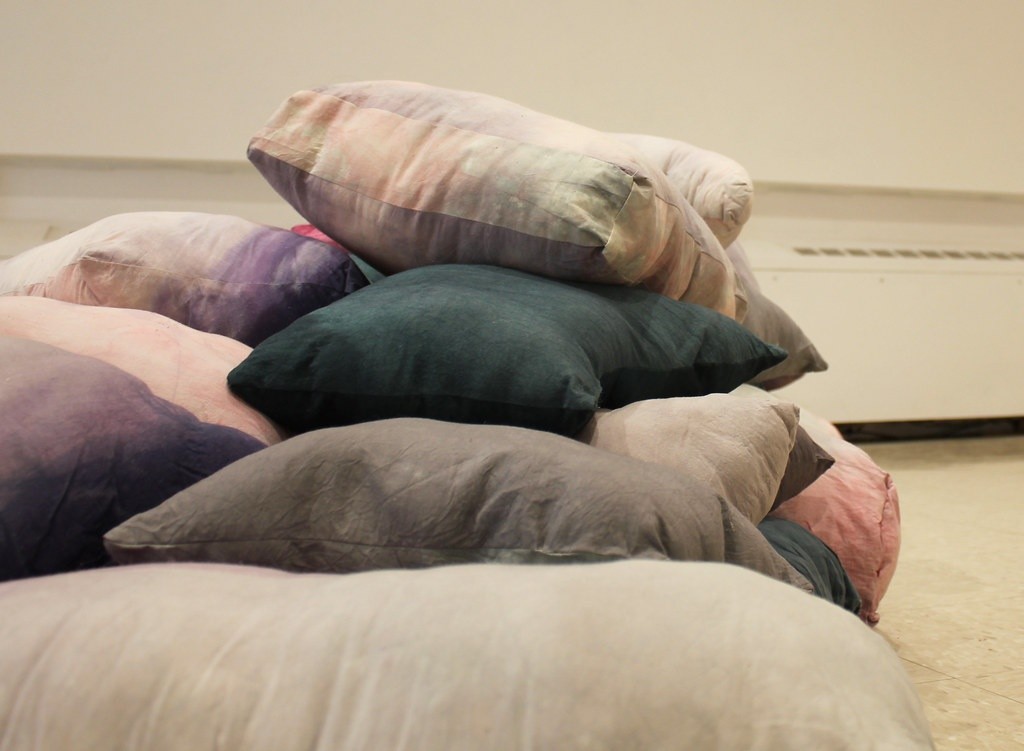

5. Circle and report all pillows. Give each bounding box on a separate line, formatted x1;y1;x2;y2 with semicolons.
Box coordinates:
247;79;829;386
101;387;901;624
0;210;370;349
0;290;281;579
228;261;788;433
